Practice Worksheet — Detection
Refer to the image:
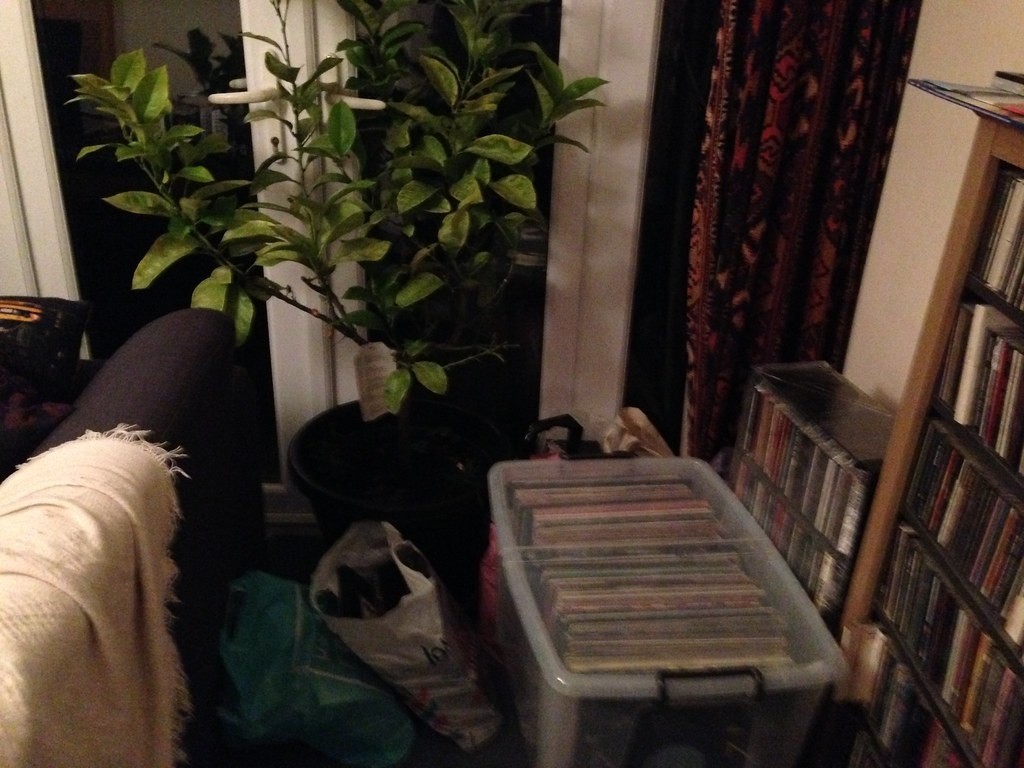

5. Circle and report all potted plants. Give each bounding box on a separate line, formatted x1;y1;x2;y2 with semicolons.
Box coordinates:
63;0;611;623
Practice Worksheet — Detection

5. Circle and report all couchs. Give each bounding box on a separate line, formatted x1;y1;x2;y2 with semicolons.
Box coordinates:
0;309;268;768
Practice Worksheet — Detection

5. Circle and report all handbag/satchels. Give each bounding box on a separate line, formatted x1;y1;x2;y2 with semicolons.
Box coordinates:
212;569;413;768
307;519;508;755
479;405;676;669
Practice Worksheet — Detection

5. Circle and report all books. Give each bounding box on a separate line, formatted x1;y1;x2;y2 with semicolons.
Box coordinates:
812;167;1021;768
731;391;852;605
495;469;824;768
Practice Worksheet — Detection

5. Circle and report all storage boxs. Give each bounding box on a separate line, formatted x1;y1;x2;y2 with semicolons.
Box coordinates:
486;456;850;768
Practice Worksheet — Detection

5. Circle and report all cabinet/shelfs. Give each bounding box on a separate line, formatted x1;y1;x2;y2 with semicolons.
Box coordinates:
842;113;1024;768
728;360;893;635
